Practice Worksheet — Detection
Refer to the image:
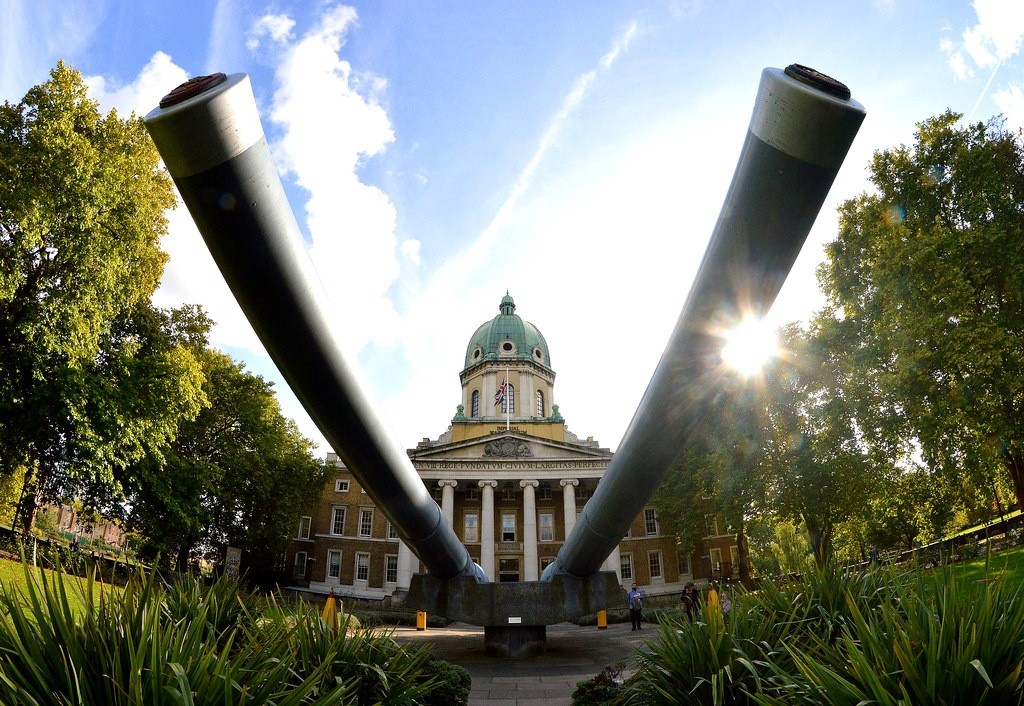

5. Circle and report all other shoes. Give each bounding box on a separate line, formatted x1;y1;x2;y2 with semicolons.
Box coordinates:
638;628;642;630
631;628;636;632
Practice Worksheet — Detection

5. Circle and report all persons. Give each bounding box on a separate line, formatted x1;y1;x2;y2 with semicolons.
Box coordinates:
627;583;645;631
722;592;732;614
70;536;79;552
681;582;701;625
868;546;880;564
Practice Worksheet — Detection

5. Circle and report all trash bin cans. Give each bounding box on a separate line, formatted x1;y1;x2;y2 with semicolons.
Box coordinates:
416;608;427;631
596;609;607;629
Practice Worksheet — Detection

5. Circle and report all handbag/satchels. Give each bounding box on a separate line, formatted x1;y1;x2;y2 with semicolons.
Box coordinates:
687;607;697;614
